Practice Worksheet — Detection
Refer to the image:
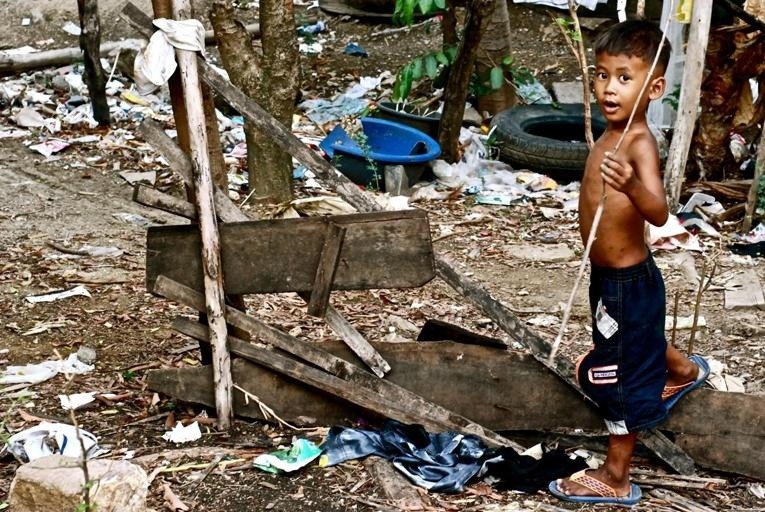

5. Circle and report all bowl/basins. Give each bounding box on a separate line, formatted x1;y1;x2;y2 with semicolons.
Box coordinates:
318;117;442;189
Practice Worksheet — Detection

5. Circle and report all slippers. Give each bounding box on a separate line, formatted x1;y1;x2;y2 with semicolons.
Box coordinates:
661;355;709;414
548;468;642;504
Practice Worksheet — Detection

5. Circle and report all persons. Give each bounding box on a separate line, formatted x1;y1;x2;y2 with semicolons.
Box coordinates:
548;18;711;505
